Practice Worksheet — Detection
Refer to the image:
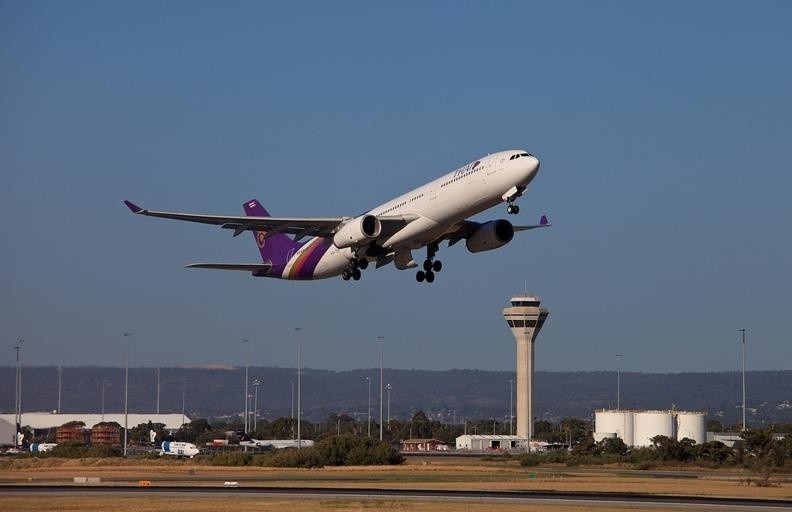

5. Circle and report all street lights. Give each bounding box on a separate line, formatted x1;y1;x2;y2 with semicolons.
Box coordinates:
616;354;623;411
360;376;372;439
11;337;25;449
123;332;133;458
384;382;392;432
101;379;108;422
240;335;263;452
376;336;385;441
735;328;746;430
508;380;516;435
295;327;303;449
154;368;161;414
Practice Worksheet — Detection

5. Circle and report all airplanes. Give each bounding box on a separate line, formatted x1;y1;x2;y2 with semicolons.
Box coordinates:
6;423;58;455
148;429;200;460
123;148;552;284
238;432;315;454
